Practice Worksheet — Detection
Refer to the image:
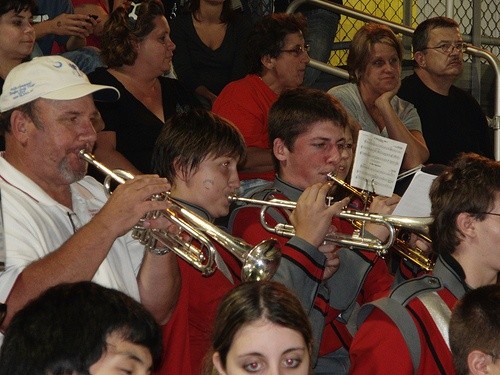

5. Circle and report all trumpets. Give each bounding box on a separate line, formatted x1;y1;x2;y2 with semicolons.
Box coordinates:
227;192;435;252
327;171;435;272
78;147;282;285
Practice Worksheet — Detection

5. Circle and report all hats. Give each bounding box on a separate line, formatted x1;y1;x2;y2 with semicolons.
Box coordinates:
0;55;121;114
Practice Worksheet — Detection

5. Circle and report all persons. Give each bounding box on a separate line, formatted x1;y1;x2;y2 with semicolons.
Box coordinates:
0;282;161;375
226;86;433;374
0;0;37;94
83;1;211;196
151;107;350;375
200;281;313;375
449;285;500;375
347;151;500;375
22;0;110;76
396;16;495;175
0;56;183;348
168;0;263;101
335;114;369;210
211;12;313;194
326;20;450;198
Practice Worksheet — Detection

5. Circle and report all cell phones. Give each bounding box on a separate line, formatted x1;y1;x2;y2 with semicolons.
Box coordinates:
85;15;98;24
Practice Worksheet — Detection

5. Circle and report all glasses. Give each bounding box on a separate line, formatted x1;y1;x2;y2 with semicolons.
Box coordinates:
280;43;311;56
419;44;466;55
